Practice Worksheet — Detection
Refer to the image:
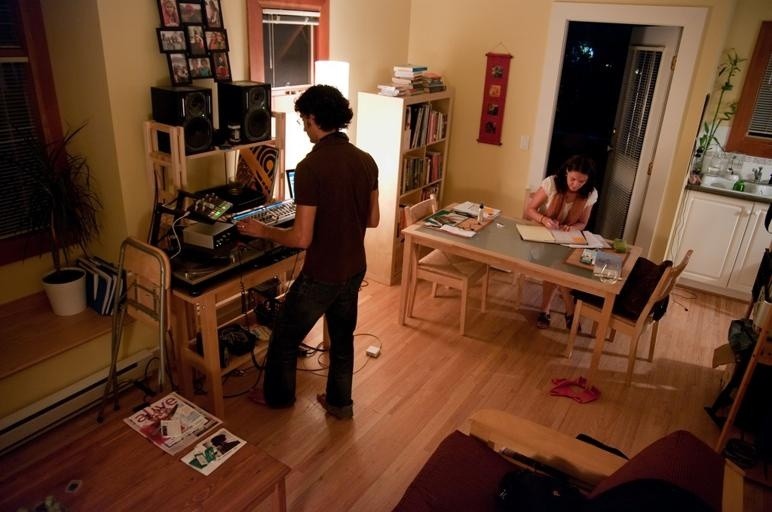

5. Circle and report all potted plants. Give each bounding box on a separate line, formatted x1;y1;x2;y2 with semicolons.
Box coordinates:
0;114;103;317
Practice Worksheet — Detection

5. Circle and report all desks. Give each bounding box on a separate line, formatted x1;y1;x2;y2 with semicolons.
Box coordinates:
172;249;331;419
0;390;292;512
399;202;643;391
714;247;772;453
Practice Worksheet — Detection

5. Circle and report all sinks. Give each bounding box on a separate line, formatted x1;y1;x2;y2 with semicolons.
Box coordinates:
706;179;772;198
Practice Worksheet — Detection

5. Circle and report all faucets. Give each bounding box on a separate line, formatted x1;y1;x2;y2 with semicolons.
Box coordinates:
752;165;763;183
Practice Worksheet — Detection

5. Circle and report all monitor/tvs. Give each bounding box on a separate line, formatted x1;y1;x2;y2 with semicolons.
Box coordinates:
286;169;296;197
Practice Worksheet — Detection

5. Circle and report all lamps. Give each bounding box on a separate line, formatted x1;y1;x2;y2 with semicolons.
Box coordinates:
315;60;349;102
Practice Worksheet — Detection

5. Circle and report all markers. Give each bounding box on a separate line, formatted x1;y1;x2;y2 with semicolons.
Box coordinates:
477;203;484;225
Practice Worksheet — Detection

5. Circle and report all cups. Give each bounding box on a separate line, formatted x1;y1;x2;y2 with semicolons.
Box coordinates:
600;263;621;286
529;242;545;260
753;298;772;329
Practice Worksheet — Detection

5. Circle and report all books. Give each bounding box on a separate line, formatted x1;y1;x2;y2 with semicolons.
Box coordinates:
377;63;448;240
74;256;126;317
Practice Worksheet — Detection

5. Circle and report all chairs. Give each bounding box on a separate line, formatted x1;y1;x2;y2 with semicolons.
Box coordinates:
392;408;744;510
564;249;693;387
97;236;172;423
404;194;489;336
512;187;543;311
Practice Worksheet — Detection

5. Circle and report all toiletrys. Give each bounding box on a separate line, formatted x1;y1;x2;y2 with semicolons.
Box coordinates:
732;179;745;191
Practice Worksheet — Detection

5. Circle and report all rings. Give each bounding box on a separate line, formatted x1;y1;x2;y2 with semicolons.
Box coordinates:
240;225;246;231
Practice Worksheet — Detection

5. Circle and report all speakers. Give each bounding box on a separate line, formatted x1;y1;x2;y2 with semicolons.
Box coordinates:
217;80;272;145
151;84;214;157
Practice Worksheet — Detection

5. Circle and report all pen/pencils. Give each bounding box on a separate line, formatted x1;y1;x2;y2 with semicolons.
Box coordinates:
550;214;559;224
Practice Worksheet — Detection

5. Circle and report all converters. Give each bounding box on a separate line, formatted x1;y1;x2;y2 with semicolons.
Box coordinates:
366;345;381;357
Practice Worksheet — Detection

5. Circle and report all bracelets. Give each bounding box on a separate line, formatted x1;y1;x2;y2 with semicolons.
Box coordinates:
536;215;544;223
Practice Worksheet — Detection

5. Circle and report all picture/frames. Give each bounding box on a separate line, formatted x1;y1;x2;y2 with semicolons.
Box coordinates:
156;0;232;86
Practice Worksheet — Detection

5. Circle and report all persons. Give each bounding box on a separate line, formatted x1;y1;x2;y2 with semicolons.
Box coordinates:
526;155;599;331
235;84;379;419
160;0;232;84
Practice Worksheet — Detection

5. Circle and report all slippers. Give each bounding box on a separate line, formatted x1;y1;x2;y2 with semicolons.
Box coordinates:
536;312;581;331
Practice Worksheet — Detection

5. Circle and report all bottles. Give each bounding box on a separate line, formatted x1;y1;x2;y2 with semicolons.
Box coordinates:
706;143;728;176
478;203;484;223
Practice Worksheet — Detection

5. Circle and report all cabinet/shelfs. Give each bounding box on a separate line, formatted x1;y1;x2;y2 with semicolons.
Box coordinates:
356;85;454;286
143;111;286;225
666;190;772;302
